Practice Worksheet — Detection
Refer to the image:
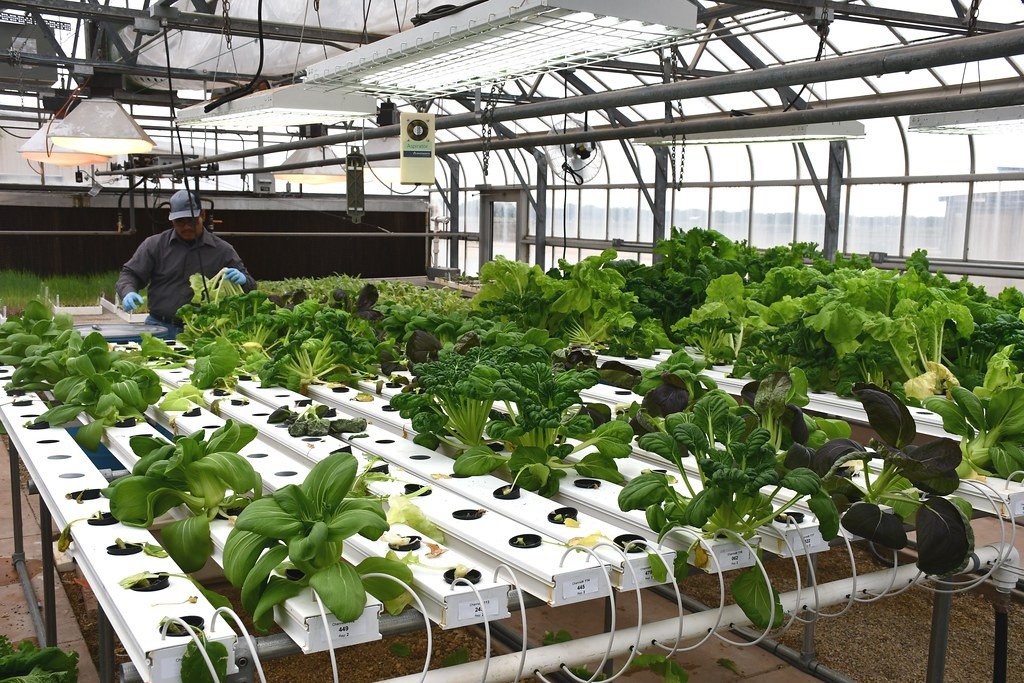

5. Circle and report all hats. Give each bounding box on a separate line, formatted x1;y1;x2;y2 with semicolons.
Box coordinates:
168;190;201;220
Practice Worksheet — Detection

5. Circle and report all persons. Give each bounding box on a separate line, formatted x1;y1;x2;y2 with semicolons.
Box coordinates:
115;190;257;340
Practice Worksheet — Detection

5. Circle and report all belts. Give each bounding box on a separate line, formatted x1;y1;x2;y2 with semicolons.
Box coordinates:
150;311;186;327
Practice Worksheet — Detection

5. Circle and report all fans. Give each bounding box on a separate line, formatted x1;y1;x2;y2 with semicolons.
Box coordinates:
545;118;603;184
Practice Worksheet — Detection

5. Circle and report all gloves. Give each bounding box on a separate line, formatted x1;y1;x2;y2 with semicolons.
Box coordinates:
223;268;246;284
122;292;144;312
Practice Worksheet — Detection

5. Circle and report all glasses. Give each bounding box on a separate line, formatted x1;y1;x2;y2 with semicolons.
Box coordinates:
173;218;198;225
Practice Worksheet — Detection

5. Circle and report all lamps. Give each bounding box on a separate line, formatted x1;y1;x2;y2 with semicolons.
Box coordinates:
16;72;157;167
